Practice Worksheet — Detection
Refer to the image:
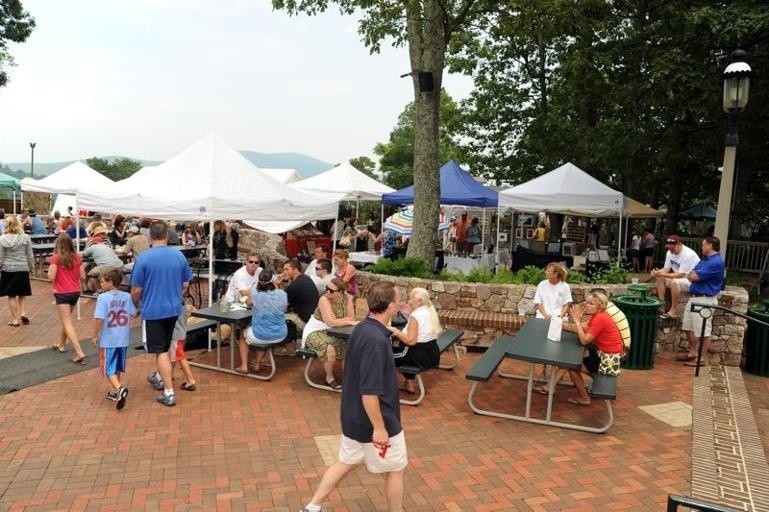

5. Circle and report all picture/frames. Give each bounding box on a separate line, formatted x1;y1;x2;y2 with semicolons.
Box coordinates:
498;233;507;242
518;215;533;227
515;228;524;238
546;241;561;253
526;229;535;239
562;242;574;257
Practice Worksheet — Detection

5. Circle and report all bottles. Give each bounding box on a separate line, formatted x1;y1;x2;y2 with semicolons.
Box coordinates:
220;294;229;315
234;289;239;303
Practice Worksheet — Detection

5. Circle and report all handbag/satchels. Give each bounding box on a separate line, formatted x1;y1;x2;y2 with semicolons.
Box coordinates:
596;350;621;376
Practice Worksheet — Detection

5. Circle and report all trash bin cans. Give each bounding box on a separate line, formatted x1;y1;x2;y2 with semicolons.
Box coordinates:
611;285;665;371
745;298;768;378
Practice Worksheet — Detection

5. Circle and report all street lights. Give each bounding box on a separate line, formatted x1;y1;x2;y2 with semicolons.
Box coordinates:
30;142;35;177
713;60;751;262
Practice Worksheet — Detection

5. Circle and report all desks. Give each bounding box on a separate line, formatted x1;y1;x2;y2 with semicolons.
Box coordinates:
443;247;511;274
349;251;382;266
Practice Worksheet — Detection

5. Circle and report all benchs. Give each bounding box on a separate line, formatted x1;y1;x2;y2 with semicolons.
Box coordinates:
398;329;465;374
186;320;216;336
295;346;317;355
590;375;617;399
466;335;514;382
249;332;301;351
30;234;247;309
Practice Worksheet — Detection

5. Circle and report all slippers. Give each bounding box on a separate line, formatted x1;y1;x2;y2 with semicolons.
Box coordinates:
53;343;67;353
659;313;681;320
533;386;555;397
72;356;88;365
325;378;343;389
180;382;196;391
567;397;589;406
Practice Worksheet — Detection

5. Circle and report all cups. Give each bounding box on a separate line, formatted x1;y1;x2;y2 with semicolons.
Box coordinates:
631;277;639;286
191;241;196;248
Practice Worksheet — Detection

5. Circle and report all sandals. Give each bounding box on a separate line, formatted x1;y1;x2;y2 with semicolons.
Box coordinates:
8;314;30;327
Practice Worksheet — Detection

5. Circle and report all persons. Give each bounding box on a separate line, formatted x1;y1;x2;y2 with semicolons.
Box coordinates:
335;205;498;257
650;233;701;319
532;216;656;274
532;261;574;329
562;288;631;394
298;280;409;512
0;207;209;411
532;292;624;406
210;251;497;395
675;236;724;367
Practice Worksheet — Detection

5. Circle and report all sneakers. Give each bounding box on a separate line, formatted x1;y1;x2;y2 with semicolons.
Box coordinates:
116;385;128;409
156;391;176;406
146;371;164;390
249;358;272;367
272;344;297;357
675;354;706;366
105;391;117;401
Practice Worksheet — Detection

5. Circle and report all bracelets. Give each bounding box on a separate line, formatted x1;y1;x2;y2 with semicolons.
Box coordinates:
576;321;581;325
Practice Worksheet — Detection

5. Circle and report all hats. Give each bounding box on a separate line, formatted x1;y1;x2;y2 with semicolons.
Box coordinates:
126;226;139;234
664;235;681;247
92;226;107;244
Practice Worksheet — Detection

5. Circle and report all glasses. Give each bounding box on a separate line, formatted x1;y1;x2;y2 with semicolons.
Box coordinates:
248;260;260;265
326;285;340;293
316;267;325;270
